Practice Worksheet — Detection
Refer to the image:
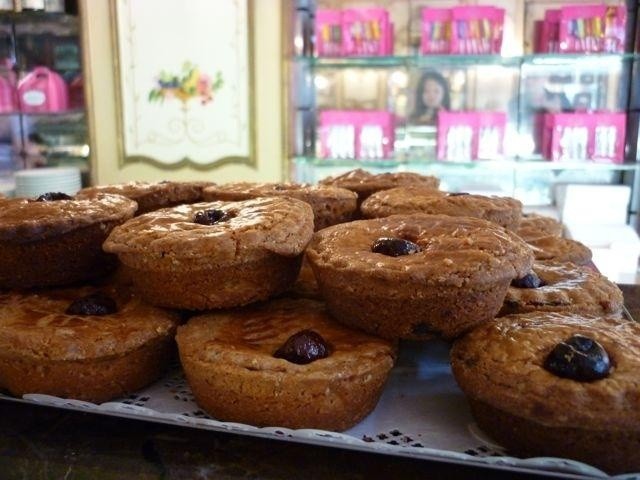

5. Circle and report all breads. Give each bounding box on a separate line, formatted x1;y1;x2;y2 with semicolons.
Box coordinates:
102;195;314;308
305;212;533;341
497;265;623;321
1;287;180;405
2;192;137;288
174;299;400;433
451;312;640;474
77;167;593;297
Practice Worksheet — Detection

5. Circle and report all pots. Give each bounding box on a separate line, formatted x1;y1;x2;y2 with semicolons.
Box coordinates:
0;0;15;12
21;0;45;11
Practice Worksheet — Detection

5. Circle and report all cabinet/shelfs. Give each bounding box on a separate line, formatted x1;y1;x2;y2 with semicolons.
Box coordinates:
1;0;93;201
280;1;638;222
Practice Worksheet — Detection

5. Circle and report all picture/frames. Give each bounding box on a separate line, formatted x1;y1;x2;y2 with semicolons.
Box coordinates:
110;0;260;169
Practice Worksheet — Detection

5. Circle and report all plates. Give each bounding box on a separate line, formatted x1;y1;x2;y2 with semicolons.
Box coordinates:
12;166;83;204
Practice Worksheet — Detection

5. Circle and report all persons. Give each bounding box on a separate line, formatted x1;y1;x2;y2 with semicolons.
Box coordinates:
407;70;452;124
510;74;570;115
